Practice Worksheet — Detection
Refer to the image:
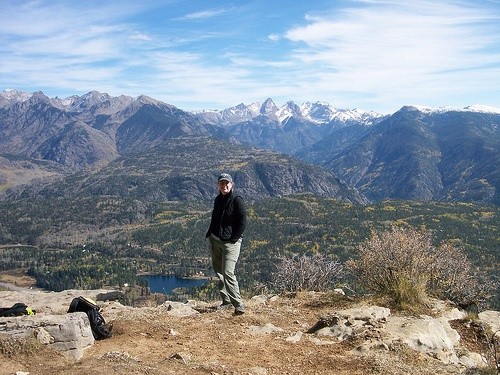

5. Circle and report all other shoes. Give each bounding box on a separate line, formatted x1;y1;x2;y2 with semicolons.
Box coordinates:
235;306;245;315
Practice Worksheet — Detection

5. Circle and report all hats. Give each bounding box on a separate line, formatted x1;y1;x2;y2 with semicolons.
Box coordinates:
218;173;233;182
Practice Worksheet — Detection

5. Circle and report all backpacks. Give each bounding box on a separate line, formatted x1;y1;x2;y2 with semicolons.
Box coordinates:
67;296;113;340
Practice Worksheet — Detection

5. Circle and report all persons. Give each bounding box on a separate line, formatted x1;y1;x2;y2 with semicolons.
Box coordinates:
206;173;247;317
